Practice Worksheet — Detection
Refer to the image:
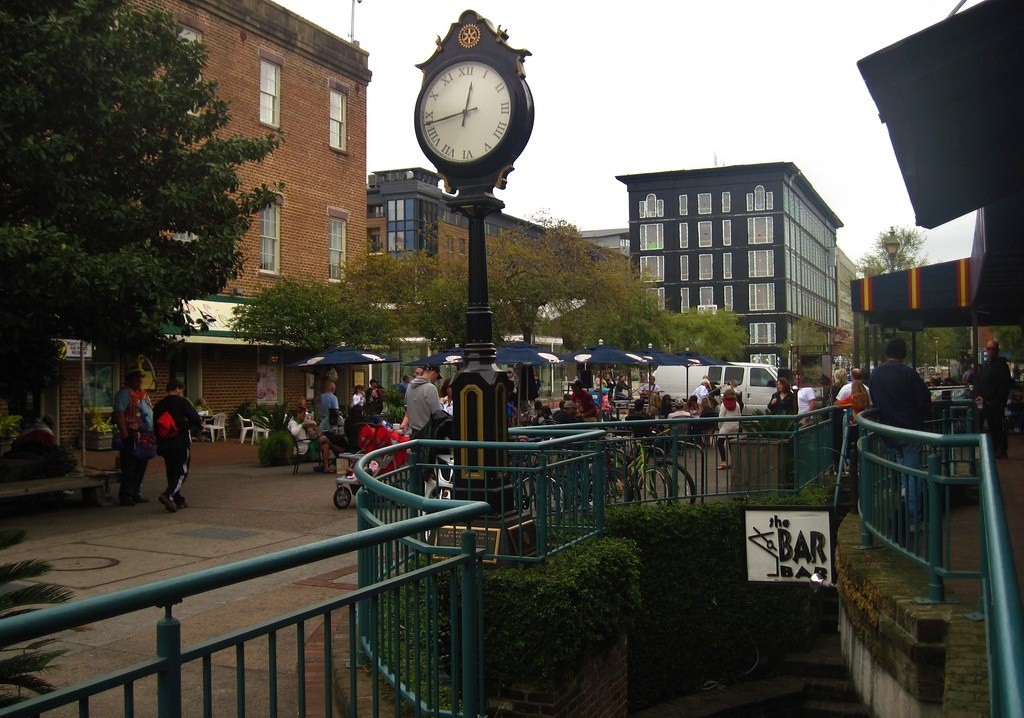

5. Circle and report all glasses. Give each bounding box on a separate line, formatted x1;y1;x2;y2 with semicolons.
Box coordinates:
986;347;997;351
776;383;782;385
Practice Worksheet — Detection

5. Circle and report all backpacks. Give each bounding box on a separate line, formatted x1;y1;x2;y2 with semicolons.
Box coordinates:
156;408;181;440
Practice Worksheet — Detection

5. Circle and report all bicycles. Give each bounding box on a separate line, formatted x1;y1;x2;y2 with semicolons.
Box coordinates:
509;429;697;513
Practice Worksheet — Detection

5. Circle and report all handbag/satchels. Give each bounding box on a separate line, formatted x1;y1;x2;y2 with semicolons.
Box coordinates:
303;422;322;440
127;428;157;459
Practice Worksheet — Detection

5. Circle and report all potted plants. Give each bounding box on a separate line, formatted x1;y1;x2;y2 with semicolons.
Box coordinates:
81;405;113;450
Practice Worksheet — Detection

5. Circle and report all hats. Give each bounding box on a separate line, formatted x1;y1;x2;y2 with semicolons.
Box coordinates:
564;401;579;408
602;387;610;394
541;405;552;415
370;378;380;384
424;363;443;380
125;369;145;378
568;379;583;387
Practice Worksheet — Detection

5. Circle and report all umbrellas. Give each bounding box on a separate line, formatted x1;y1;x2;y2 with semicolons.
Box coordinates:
494;341;733;426
403;347;466;365
284;341;402;419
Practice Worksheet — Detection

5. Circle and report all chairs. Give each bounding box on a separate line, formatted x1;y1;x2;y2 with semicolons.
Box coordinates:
238;413;352;475
201;413;227;442
542;412;718;459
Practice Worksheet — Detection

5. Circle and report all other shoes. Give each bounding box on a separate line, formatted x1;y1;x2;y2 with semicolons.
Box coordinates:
121;496;150;507
323;469;333;473
159;495;177;512
332;447;345;453
177;500;189;508
910;520;924;531
993;450;1008;459
718;463;726;470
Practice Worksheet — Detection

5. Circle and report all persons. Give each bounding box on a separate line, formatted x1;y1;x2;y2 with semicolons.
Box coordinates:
112;367;155;504
822;340;1024;474
768;377;799;416
506;371;743;470
287;364;453;480
152;379;203;513
11;414;74;496
868;339;931;532
194;398;218;441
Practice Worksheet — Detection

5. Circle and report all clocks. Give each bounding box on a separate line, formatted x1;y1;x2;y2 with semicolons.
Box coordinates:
413;10;535;193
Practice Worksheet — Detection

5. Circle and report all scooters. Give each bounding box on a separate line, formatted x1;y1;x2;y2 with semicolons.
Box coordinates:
421;449;454;544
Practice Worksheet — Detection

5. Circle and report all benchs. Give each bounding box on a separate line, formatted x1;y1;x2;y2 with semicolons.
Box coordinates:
0;476;105;507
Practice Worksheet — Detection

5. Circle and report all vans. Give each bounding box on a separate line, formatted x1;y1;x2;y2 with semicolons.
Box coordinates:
652;362;794;427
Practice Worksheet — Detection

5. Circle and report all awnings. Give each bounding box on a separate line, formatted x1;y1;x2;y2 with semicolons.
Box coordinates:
856;1;1024;229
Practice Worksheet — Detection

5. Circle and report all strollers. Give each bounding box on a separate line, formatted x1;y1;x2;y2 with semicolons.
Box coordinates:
332;426;413;509
